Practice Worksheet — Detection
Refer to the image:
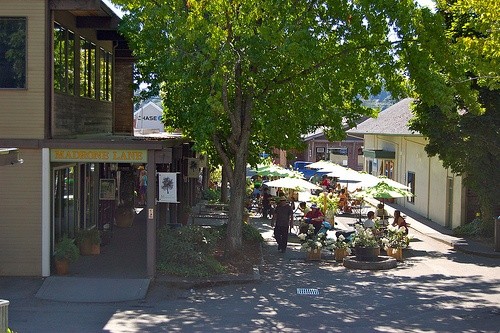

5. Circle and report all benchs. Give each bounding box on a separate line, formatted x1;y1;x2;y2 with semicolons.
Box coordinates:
191;204;228;224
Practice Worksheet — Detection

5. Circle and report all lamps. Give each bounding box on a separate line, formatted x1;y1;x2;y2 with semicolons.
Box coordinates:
8;150;24;165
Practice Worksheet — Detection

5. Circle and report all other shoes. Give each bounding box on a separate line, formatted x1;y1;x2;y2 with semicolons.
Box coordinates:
278;245;286;254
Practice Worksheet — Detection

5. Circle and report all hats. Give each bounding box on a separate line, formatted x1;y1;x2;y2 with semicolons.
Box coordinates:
280;195;287;201
310;204;319;209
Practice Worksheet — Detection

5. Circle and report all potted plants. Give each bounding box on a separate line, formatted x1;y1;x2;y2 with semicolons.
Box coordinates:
243;224;264;243
55;232;81;276
117;184;135;231
80;229;102;256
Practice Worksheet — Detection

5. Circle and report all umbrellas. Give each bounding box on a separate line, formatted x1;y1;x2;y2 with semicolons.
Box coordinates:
304;159;411;204
355;180;416;230
250;161;322;197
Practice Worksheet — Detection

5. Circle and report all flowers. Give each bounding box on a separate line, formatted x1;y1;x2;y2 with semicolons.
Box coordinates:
298;224;409;253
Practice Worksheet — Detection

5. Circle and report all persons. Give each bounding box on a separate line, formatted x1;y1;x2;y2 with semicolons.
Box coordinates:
117;163;147;206
253;176;295;218
338;189;346;211
271;196;293;253
309;173;319;191
350;188;362;212
364;211;375;230
298;202;324;235
375;204;388;227
321;175;330;186
392;210;408;236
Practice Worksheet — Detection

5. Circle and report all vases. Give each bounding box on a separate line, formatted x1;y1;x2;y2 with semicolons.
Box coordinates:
335;248;349;260
387;247;403;261
356;246;381;256
308;248;321;259
